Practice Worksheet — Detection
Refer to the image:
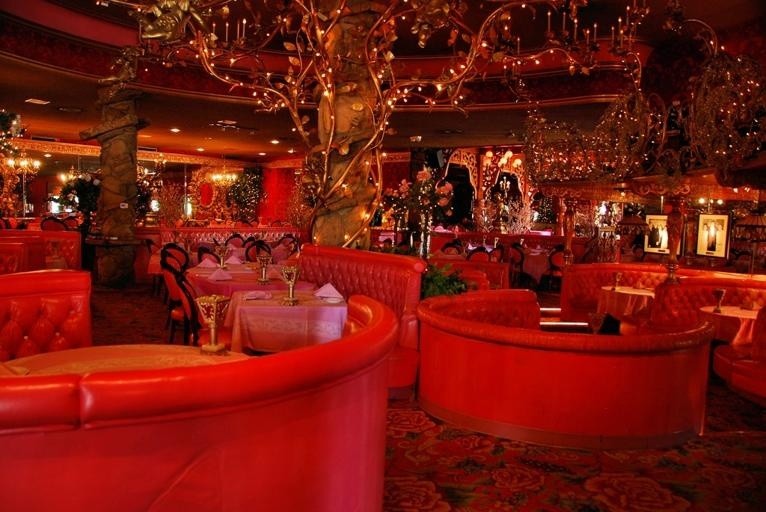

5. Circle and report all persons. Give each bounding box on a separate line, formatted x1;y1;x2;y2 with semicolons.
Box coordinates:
701;220;724;252
648;222;669;248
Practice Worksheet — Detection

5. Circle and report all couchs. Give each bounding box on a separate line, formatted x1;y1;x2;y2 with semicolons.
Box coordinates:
416;233;762;449
0;217;426;510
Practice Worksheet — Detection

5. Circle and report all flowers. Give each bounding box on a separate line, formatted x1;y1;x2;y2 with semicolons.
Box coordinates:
378;167;456;214
